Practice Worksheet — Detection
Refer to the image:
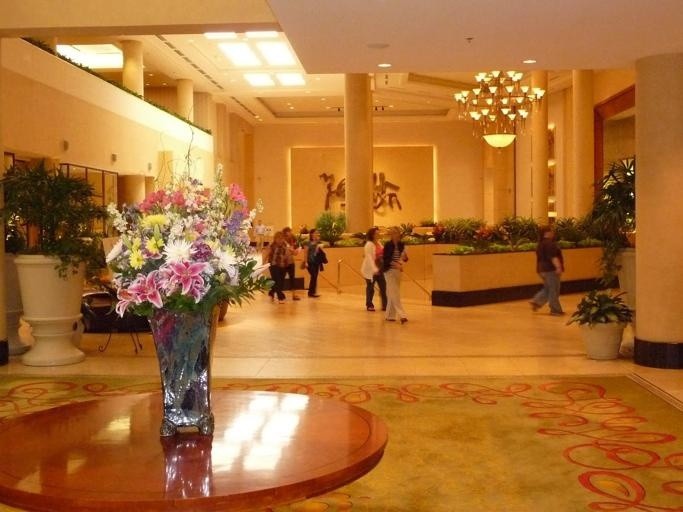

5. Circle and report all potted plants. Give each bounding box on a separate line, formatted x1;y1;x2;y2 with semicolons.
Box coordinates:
0;155;114;368
565;289;636;359
578;154;636;333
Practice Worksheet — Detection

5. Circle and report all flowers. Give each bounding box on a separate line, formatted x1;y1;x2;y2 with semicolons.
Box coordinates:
103;102;277;320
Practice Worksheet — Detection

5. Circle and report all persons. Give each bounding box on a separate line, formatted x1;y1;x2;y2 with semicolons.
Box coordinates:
267;232;288;304
281;227;300;300
360;226;388;311
528;225;567;316
303;228;324;298
296;224;309;250
381;225;409;325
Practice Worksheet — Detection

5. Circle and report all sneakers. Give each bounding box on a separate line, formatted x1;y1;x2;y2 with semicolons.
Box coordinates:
267;292;321;305
528;298;567;316
363;304;409;324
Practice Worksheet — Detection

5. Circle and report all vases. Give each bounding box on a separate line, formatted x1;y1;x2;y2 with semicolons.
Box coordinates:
146;308;214;437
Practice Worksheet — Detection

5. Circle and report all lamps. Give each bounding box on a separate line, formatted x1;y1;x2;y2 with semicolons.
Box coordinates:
453;69;547;150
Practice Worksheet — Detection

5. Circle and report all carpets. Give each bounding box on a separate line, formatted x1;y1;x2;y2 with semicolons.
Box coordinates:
0;371;683;512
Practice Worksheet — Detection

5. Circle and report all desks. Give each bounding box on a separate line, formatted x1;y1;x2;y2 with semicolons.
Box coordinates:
1;390;388;511
